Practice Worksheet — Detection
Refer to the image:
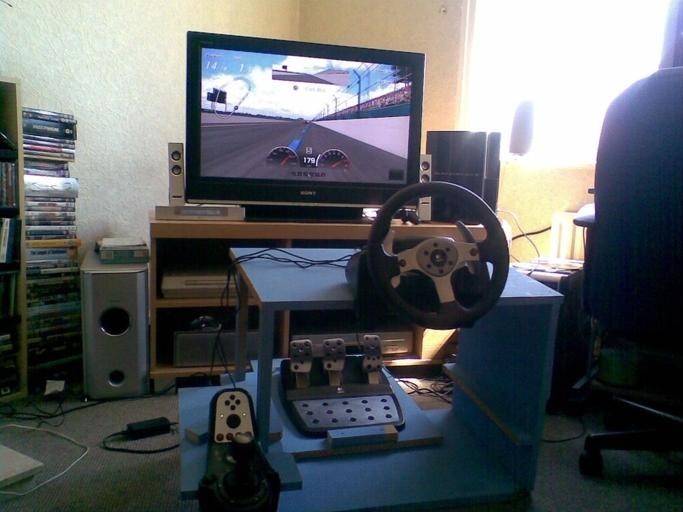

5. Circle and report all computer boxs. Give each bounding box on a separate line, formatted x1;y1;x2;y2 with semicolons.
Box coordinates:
510;256;584;414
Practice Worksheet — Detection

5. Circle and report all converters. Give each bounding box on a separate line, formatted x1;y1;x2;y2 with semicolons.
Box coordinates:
126;417;170;440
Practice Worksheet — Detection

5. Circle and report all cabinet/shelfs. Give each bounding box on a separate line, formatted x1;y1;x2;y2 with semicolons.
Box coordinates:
0;75;31;408
147;209;490;398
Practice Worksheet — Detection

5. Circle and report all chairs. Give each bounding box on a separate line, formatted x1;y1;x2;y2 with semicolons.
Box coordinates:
570;65;681;473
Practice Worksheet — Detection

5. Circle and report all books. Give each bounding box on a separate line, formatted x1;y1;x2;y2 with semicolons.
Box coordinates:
0;107;82;373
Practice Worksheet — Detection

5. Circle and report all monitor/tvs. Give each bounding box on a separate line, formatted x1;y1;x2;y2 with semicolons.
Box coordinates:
184;30;425;210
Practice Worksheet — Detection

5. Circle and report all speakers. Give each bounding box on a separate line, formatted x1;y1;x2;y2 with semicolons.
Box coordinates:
426;131;501;225
417;154;431;221
168;143;184;206
80;249;151;399
